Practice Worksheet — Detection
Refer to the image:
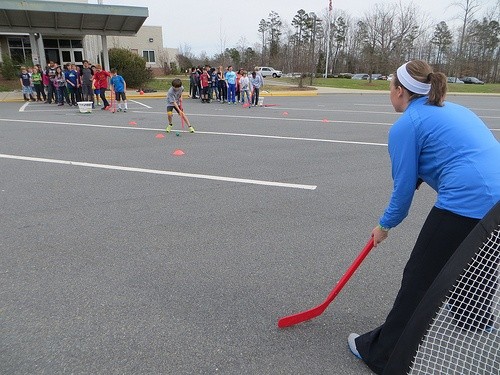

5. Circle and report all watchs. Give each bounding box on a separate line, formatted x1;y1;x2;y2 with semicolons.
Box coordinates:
379;224;389;231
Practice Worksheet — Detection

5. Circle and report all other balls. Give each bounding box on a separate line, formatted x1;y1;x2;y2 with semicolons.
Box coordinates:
176;131;180;136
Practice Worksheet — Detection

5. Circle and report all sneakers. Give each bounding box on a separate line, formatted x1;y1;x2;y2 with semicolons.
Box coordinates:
446;299;493;331
167;125;172;133
188;127;194;133
348;333;368;363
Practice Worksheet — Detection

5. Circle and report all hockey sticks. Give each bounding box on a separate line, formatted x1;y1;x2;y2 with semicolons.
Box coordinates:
241;87;254;109
260;87;272;96
180;100;186;134
110;94;116;112
105;88;113;110
278;179;423;328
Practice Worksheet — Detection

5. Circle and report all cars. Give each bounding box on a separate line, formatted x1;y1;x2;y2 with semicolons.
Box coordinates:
352;74;369;80
367;74;383;80
387;74;393;80
447;76;464;84
461;77;485;85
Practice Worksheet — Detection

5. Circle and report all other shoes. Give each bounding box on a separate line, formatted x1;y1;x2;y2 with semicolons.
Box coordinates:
68;103;72;106
117;108;122;112
124;108;127;112
217;99;220;102
228;102;230;105
75;104;77;106
58;103;62;106
92;107;95;110
234;102;237;104
256;105;257;107
101;104;110;110
210;99;213;102
97;103;101;106
252;104;254;107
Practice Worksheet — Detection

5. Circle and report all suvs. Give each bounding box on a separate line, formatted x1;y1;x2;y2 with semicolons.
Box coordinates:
258;67;283;78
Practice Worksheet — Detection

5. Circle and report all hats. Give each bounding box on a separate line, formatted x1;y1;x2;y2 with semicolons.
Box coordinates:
251;72;256;75
111;68;116;73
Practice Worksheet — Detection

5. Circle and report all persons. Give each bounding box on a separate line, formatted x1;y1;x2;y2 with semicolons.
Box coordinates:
346;60;500;375
19;60;102;106
109;68;128;112
166;79;194;133
90;64;112;110
188;65;264;108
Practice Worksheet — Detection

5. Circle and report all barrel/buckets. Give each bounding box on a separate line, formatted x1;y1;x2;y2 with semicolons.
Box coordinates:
254;97;264;105
77;101;93;113
